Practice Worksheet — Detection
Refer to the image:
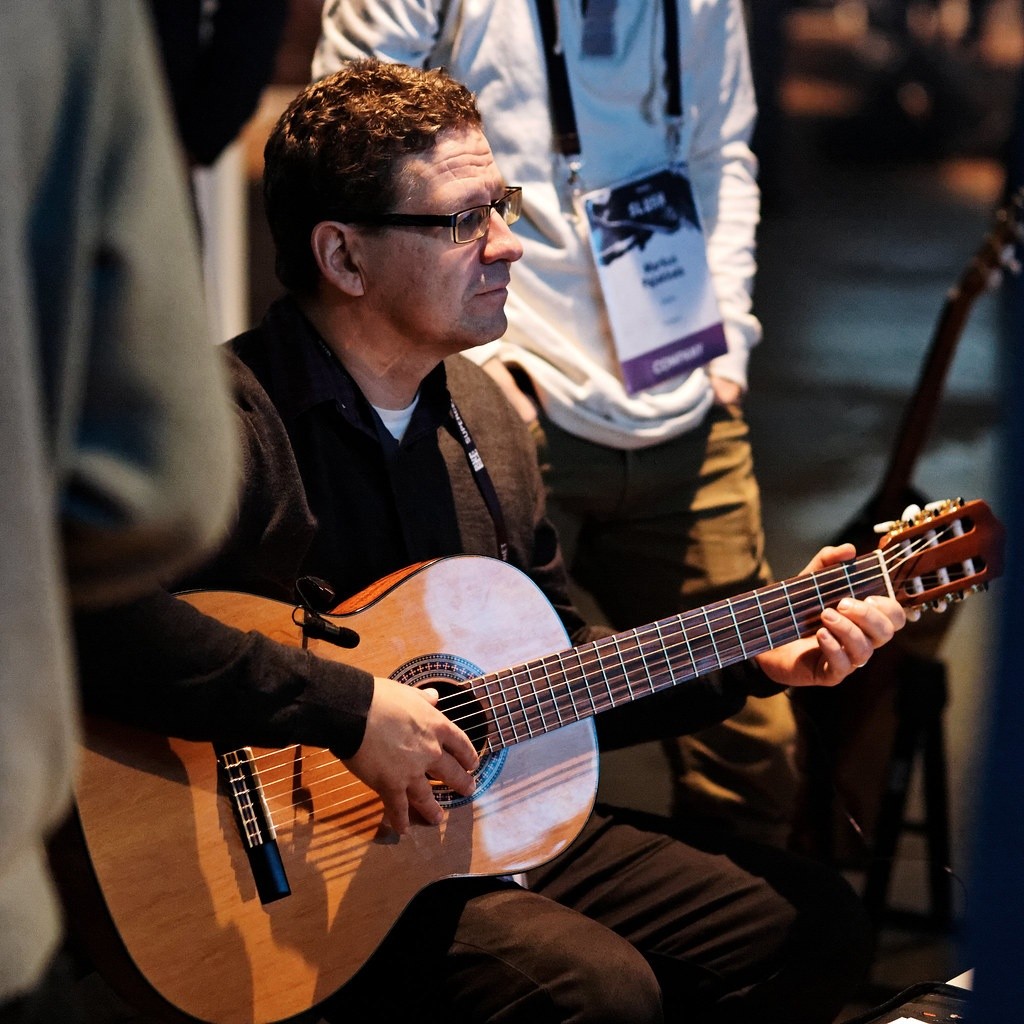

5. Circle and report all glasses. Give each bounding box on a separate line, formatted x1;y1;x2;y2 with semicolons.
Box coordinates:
347;186;522;244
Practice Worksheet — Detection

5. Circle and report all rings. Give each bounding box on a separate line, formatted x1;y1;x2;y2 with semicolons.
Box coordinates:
851;661;869;667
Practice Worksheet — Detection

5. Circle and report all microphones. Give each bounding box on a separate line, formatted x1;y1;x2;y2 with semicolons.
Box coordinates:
302;617;361;648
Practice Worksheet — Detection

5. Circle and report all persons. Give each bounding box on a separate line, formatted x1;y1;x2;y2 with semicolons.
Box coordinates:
1;0;1024;1024
70;57;907;1024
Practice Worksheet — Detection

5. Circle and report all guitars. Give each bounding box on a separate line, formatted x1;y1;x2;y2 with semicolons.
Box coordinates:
69;491;1003;1023
828;176;1021;657
